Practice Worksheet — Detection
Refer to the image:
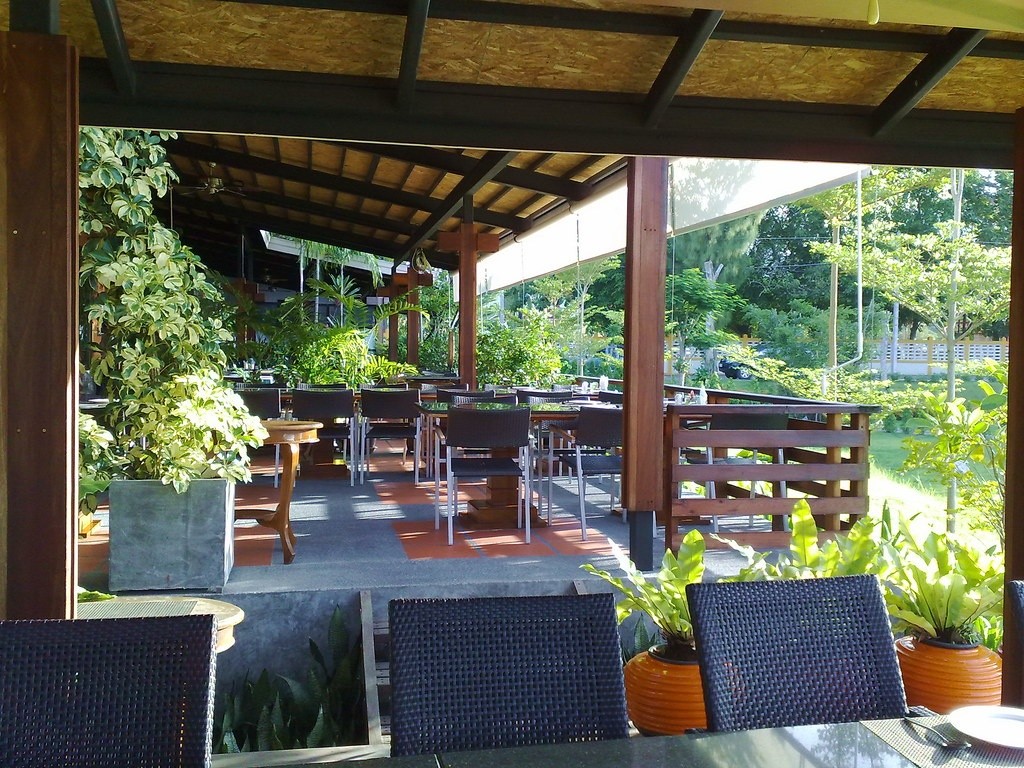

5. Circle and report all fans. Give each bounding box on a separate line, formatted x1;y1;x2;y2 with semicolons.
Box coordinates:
175;159;246;200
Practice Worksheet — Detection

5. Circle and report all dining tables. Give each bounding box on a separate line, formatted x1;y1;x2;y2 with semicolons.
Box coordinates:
305;714;1024;768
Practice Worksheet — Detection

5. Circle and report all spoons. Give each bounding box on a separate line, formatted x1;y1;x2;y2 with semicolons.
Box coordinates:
903;716;966;751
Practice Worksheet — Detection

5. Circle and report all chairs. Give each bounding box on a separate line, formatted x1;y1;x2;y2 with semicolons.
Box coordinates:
683;574;909;730
77;370;798;554
0;613;219;768
387;592;632;754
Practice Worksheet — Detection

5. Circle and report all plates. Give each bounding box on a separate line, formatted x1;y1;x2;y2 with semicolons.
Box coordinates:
948;706;1024;748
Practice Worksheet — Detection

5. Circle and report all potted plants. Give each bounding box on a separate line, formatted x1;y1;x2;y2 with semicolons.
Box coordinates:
102;365;272;595
884;534;1002;711
576;529;711;736
